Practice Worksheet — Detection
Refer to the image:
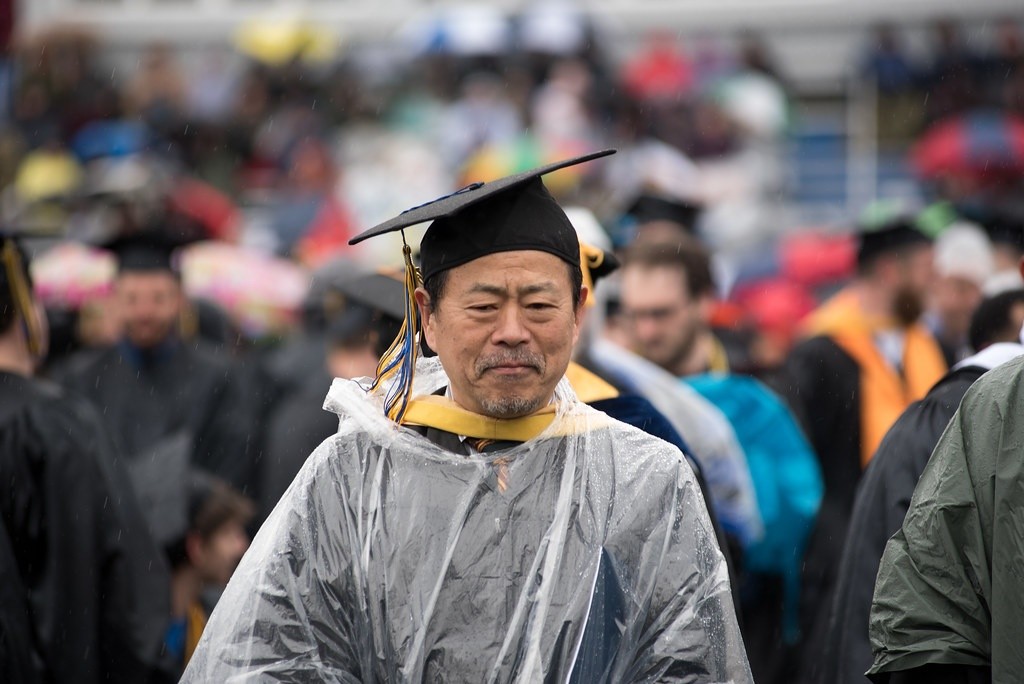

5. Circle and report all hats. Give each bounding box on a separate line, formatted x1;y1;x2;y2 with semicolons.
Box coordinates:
852;215;932;260
333;274;405;345
0;228;64;360
83;228;213;343
124;428;213;553
348;147;619;424
619;188;703;232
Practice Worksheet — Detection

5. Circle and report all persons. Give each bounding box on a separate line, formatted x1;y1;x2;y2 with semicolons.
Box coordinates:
267;295;412;530
813;330;1024;684
780;212;952;595
1;0;1024;420
863;255;1024;684
178;191;758;684
154;485;249;683
600;242;824;649
548;202;764;615
0;237;181;684
65;246;268;684
